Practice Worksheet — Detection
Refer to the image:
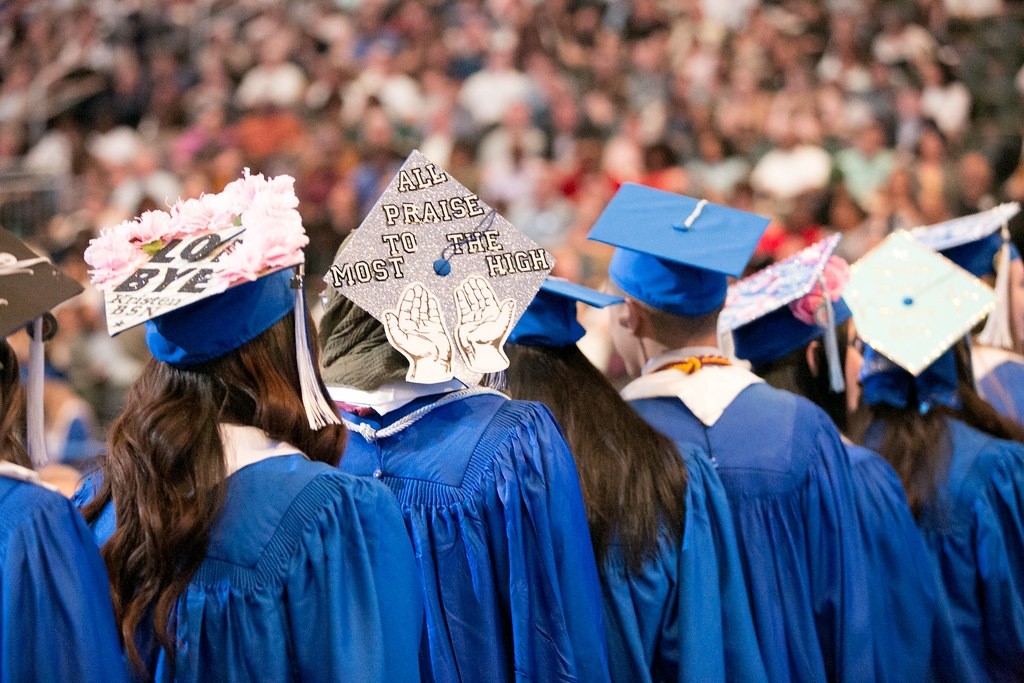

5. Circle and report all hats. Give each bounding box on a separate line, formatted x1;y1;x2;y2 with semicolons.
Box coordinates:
837;226;1002;408
723;232;854;369
911;201;1024;278
82;169;342;430
508;275;626;350
0;224;85;466
590;183;772;320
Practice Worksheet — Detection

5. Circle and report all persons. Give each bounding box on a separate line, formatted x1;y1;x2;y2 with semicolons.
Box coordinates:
844;229;1024;683
717;231;975;683
479;275;769;683
589;181;875;683
315;150;611;683
913;202;1024;425
0;227;132;683
70;172;423;683
0;0;1024;500
